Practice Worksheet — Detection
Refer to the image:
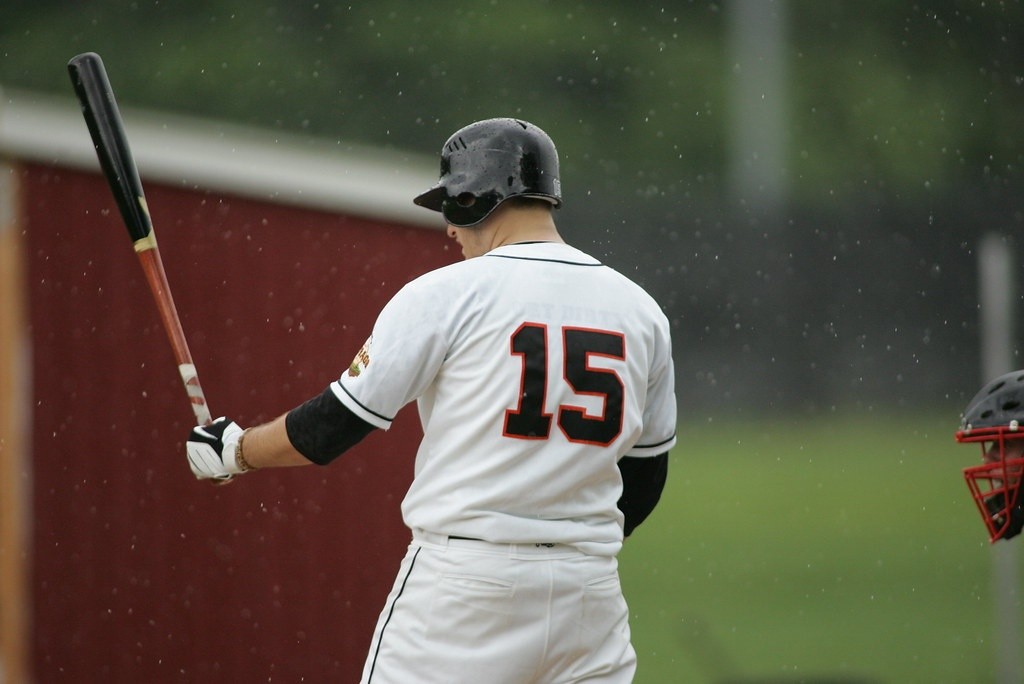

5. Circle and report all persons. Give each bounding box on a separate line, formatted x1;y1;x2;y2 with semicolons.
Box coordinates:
953;370;1024;542
186;117;677;684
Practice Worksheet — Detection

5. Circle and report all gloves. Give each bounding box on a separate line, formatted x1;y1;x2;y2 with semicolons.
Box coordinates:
186;416;249;480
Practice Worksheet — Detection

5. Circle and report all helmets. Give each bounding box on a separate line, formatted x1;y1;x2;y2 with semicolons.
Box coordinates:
413;118;562;228
955;370;1024;540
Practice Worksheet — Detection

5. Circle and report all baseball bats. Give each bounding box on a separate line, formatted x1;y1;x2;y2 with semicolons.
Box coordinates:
68;53;235;488
